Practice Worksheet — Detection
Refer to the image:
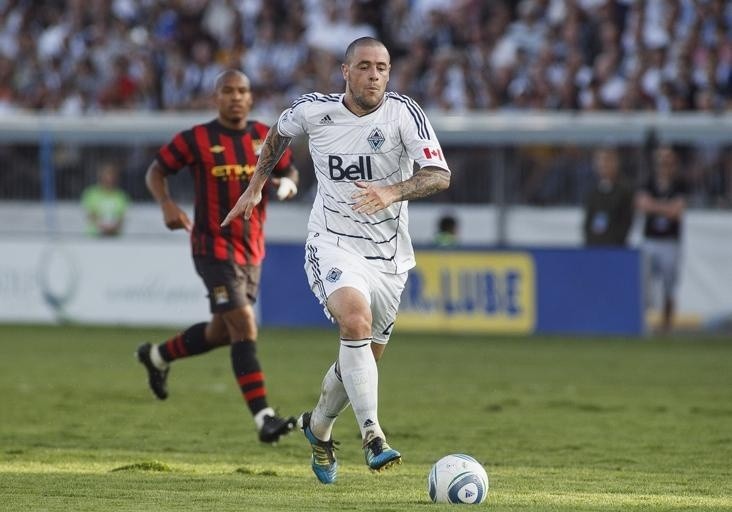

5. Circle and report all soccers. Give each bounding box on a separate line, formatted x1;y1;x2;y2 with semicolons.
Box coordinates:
427;453;489;504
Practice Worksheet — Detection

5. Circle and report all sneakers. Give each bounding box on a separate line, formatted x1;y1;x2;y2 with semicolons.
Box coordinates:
138;342;170;399
364;435;402;474
259;413;297;443
296;410;337;484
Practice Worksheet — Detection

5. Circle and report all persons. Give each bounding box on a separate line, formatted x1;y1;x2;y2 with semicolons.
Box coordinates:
580;146;637;247
630;139;689;336
219;36;452;485
78;156;136;237
134;67;301;444
431;215;461;246
1;0;732;208
